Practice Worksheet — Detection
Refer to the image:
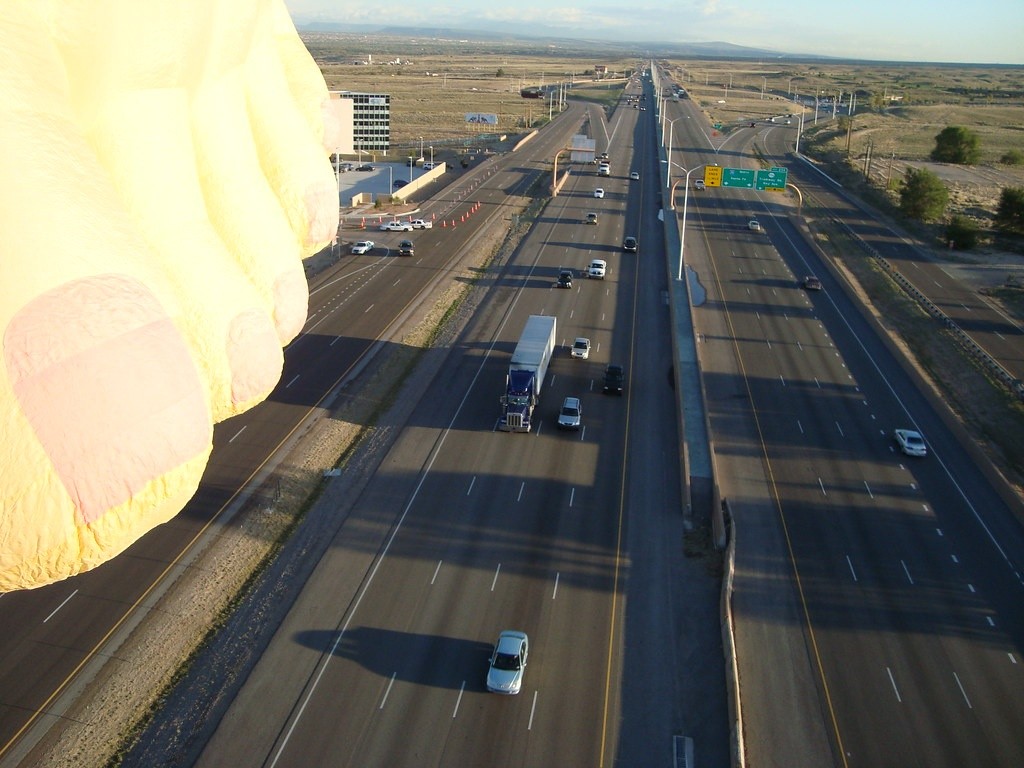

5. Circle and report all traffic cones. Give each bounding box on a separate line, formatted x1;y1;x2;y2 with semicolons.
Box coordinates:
360;166;498;227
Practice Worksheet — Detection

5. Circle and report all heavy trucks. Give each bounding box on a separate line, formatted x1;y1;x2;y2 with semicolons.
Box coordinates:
497;314;557;433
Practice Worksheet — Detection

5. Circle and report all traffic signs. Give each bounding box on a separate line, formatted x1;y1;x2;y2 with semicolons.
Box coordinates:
722;168;786;192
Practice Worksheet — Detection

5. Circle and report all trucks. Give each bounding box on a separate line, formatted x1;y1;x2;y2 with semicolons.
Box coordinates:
598;161;611;177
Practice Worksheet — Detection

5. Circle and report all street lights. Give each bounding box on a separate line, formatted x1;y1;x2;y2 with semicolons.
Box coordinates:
336;136;433;182
389;166;392;193
661;160;717;279
654;95;675;148
656;114;691;188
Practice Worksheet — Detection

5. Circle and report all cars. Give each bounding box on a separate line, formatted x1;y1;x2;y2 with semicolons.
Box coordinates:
351;219;432;257
485;630;529;696
694;180;706;191
895;429;926;457
588;96;647;165
748;220;760;230
803;275;821;289
558;338;624;429
333;160;436;188
557;172;639;289
749;118;791;128
